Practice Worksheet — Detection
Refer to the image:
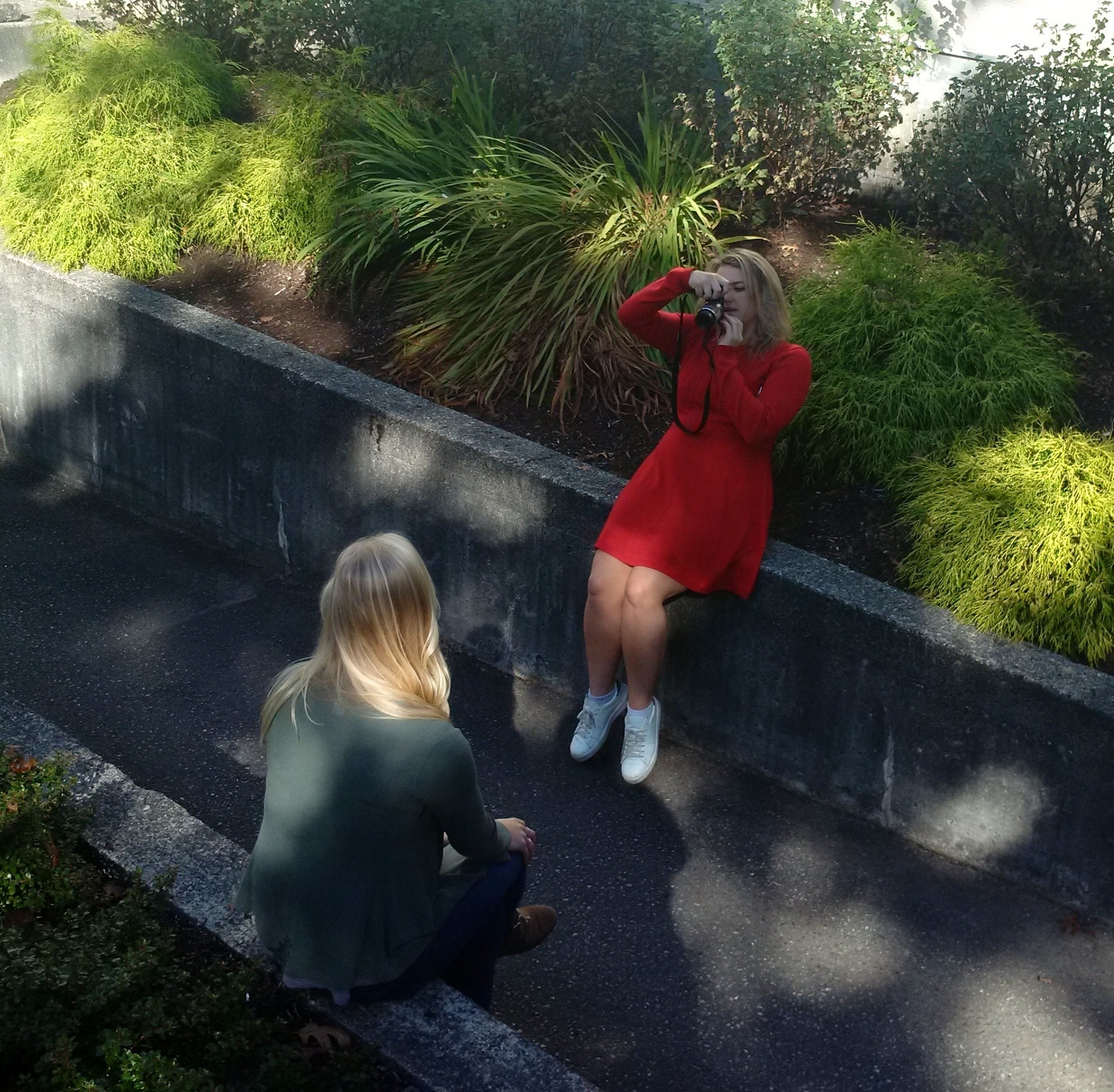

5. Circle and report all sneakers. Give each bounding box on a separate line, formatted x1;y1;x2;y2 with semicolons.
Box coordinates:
570;680;628;761
621;696;660;784
496;905;557;959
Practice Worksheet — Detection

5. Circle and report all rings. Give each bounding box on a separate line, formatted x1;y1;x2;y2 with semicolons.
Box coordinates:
526;837;532;845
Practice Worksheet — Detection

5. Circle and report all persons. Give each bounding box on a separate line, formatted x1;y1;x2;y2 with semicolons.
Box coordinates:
232;534;557;1010
570;248;812;783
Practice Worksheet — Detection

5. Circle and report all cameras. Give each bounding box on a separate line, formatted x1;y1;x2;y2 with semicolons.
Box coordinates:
695;285;725;330
